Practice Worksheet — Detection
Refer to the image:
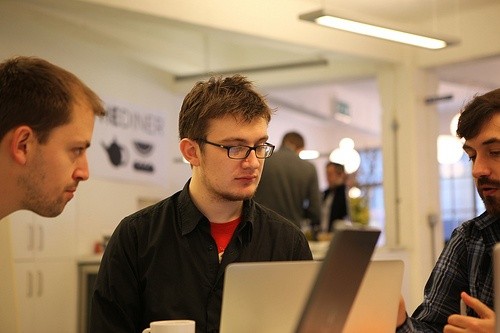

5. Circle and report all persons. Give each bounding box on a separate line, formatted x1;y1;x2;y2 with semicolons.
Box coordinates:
252;131;320;233
0;57;106;221
395;87;500;333
320;161;368;235
86;74;313;333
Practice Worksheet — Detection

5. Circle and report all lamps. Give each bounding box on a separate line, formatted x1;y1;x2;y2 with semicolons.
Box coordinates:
173;29;328;83
299;0;460;52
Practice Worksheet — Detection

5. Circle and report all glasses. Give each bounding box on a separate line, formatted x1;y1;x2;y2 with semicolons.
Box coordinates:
193;137;275;159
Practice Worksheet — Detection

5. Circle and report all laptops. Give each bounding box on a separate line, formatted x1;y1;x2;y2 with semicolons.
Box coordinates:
220;259;403;333
296;227;382;333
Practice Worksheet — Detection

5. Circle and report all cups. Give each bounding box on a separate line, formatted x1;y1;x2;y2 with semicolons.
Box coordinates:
142;320;195;333
